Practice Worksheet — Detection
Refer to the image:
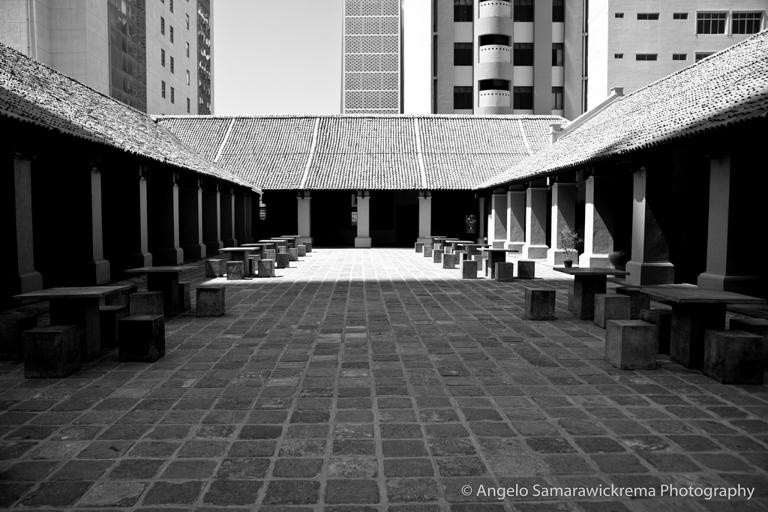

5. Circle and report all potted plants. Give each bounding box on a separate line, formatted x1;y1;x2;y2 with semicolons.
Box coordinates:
559;224;583;268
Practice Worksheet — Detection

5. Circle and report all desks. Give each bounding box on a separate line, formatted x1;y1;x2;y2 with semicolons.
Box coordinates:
424;235;475;264
258;235;301;261
